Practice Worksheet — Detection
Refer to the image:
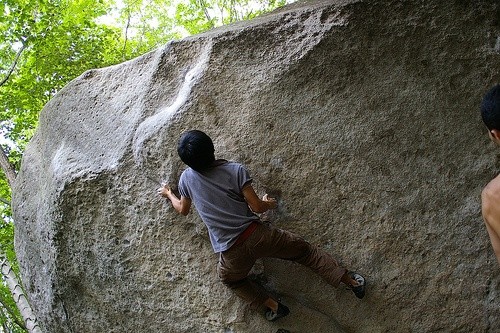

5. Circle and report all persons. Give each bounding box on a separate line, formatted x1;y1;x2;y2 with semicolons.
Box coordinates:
155;128;367;322
477;81;500;268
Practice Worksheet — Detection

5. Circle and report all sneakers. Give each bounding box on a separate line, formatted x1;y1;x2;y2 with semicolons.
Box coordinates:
349;274;367;299
264;302;290;323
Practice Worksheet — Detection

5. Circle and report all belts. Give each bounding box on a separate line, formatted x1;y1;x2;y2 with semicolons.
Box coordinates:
222;220;261;253
